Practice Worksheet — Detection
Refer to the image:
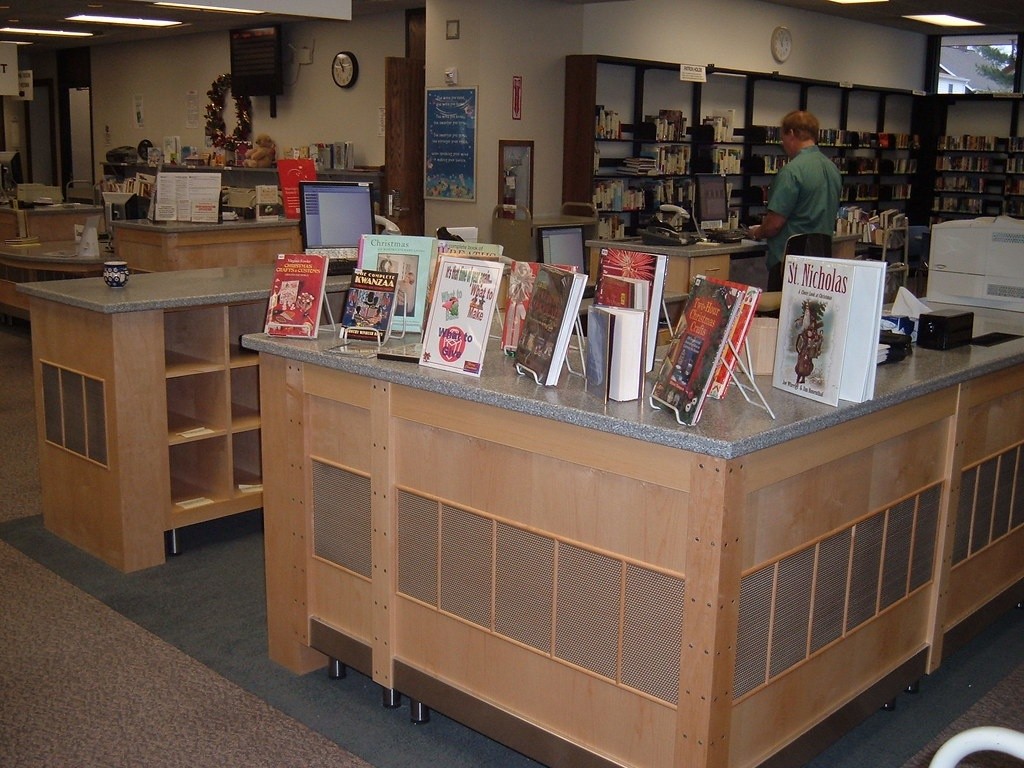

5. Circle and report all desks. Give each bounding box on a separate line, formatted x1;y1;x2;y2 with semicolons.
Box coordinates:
585;227;864;295
99;161;386;201
241;300;1023;768
109;215;304;276
0;241;120;327
0;203;104;242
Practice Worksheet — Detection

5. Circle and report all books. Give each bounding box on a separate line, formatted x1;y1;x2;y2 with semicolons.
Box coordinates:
587;248;670;404
264;254;329;339
148;136;181;166
593;104;696;239
256;159;316;220
703;109;793;206
652;275;761;427
283;141;355;169
340;268;399;343
772;255;887;408
818;128;920;200
929;134;1024;230
357;233;504;377
847;207;905;303
501;261;588;387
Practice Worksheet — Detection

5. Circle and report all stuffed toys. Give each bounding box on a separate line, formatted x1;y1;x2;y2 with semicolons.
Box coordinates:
243;135;275;168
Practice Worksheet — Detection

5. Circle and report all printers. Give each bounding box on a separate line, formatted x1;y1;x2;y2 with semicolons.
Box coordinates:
927;216;1024;314
16;183;64;205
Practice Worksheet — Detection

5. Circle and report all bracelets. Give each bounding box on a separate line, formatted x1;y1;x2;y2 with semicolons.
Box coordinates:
752;229;760;241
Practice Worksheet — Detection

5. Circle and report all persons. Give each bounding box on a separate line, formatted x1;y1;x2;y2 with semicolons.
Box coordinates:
747;111;842;291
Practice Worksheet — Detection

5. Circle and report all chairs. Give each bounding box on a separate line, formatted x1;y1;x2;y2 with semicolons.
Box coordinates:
754;234;832;319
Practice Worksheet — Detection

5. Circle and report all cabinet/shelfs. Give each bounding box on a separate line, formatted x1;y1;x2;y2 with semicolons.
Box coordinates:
492;201;598;273
922;91;1024;227
67;179;103;206
857;227;908;303
564;53;922;238
16;264;352;574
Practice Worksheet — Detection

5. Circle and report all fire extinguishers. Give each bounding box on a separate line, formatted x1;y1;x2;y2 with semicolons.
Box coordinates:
502;164;521;219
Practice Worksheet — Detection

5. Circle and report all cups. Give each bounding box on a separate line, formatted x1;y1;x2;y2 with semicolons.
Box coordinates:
103;261;130;288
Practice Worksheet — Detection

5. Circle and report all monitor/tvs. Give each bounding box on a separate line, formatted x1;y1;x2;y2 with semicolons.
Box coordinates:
688;173;729;232
102;192;140;252
0;152;23;203
299;180;376;251
230;23;283;95
536;225;587;274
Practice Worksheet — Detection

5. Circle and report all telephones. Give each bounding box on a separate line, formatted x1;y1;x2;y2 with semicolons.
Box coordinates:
641;226;688;245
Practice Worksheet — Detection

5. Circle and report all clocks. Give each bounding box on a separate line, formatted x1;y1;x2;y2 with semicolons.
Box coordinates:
771;26;793;63
332;52;359;88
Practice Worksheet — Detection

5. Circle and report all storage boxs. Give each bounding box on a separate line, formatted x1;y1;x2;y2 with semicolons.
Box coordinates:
926;216;1024;313
916;308;974;350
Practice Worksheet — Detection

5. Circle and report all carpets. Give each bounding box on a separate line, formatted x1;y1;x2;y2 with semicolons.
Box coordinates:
0;512;1024;768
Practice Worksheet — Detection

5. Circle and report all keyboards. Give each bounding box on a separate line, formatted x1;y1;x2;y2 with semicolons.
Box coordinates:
730;230;747;239
